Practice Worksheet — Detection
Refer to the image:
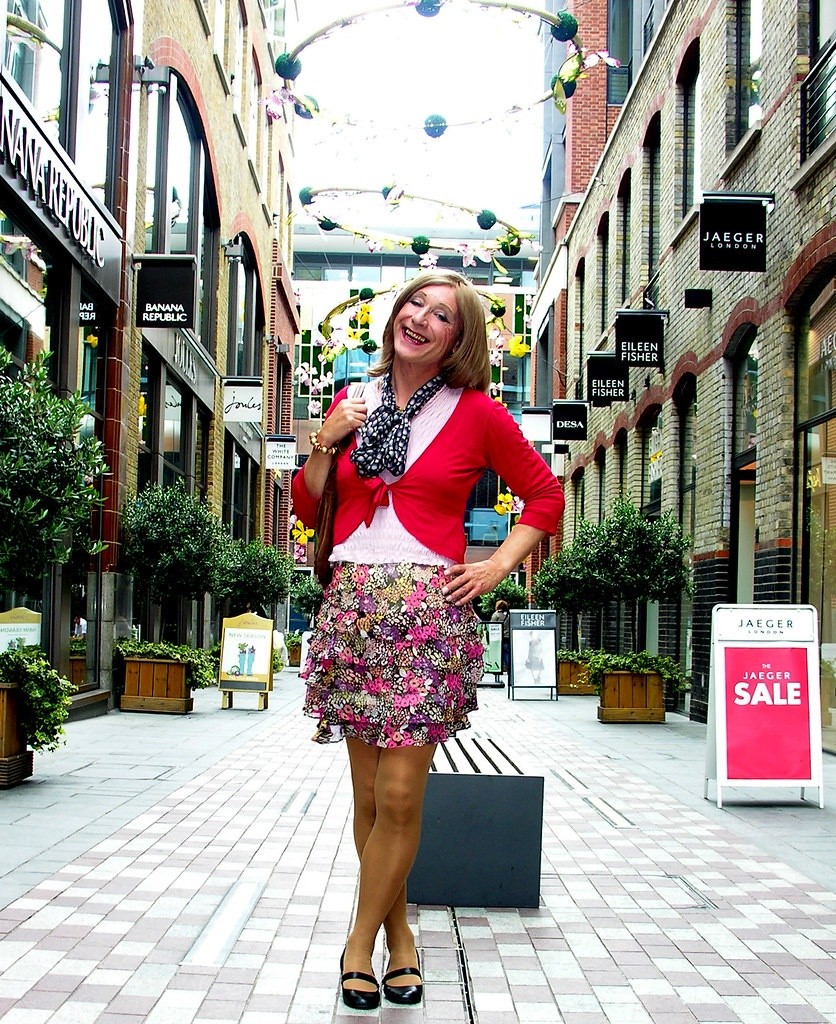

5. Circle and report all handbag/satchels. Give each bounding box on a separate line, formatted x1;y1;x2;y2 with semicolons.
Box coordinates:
312;461;338;588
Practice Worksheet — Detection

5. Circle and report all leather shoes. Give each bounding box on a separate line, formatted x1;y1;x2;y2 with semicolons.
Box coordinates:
382;950;423;1004
340;946;380;1008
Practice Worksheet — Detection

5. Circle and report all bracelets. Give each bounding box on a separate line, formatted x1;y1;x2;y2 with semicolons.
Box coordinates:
306;429;338;454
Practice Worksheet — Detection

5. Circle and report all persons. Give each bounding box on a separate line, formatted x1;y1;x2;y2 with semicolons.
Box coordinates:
72;612;87;640
489;598;510;674
527;631;545;683
290;264;576;1013
